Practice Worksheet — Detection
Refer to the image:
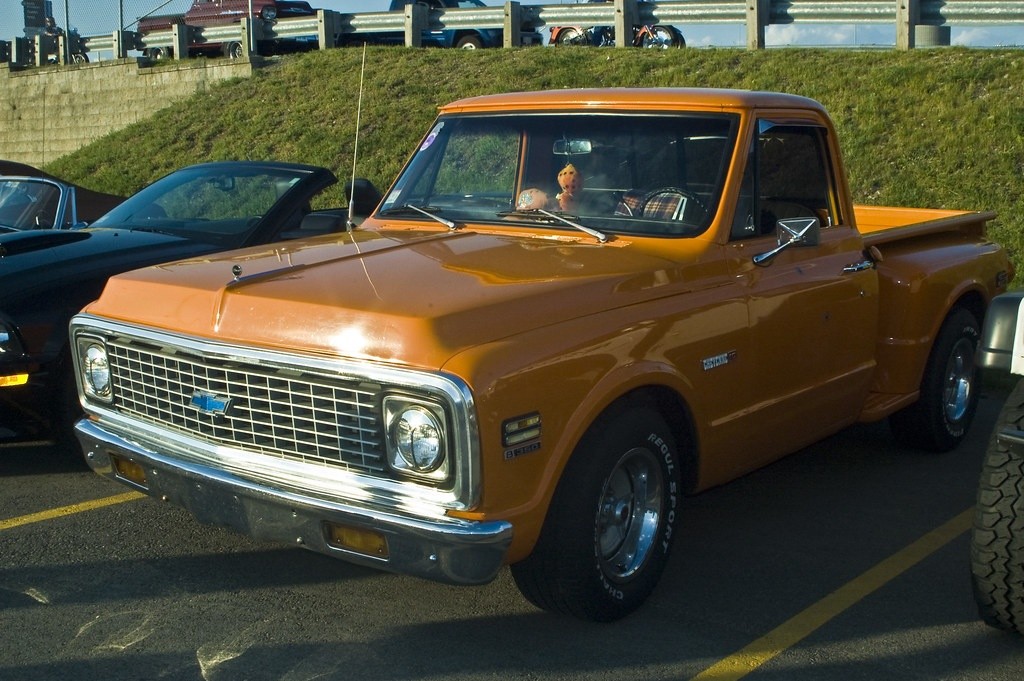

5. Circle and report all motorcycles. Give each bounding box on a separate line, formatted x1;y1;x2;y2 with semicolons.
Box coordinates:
548;0;686;52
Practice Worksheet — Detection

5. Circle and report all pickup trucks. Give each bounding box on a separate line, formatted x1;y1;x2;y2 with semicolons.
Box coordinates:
70;87;1016;624
341;0;504;49
136;0;322;63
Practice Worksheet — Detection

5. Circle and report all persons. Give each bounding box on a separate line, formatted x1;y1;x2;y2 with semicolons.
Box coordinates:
45;17;62;36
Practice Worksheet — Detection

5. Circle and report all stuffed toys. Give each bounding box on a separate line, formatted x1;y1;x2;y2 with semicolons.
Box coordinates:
556;165;584;212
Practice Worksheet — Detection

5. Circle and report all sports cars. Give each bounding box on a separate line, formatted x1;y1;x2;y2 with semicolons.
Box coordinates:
0;160;471;461
